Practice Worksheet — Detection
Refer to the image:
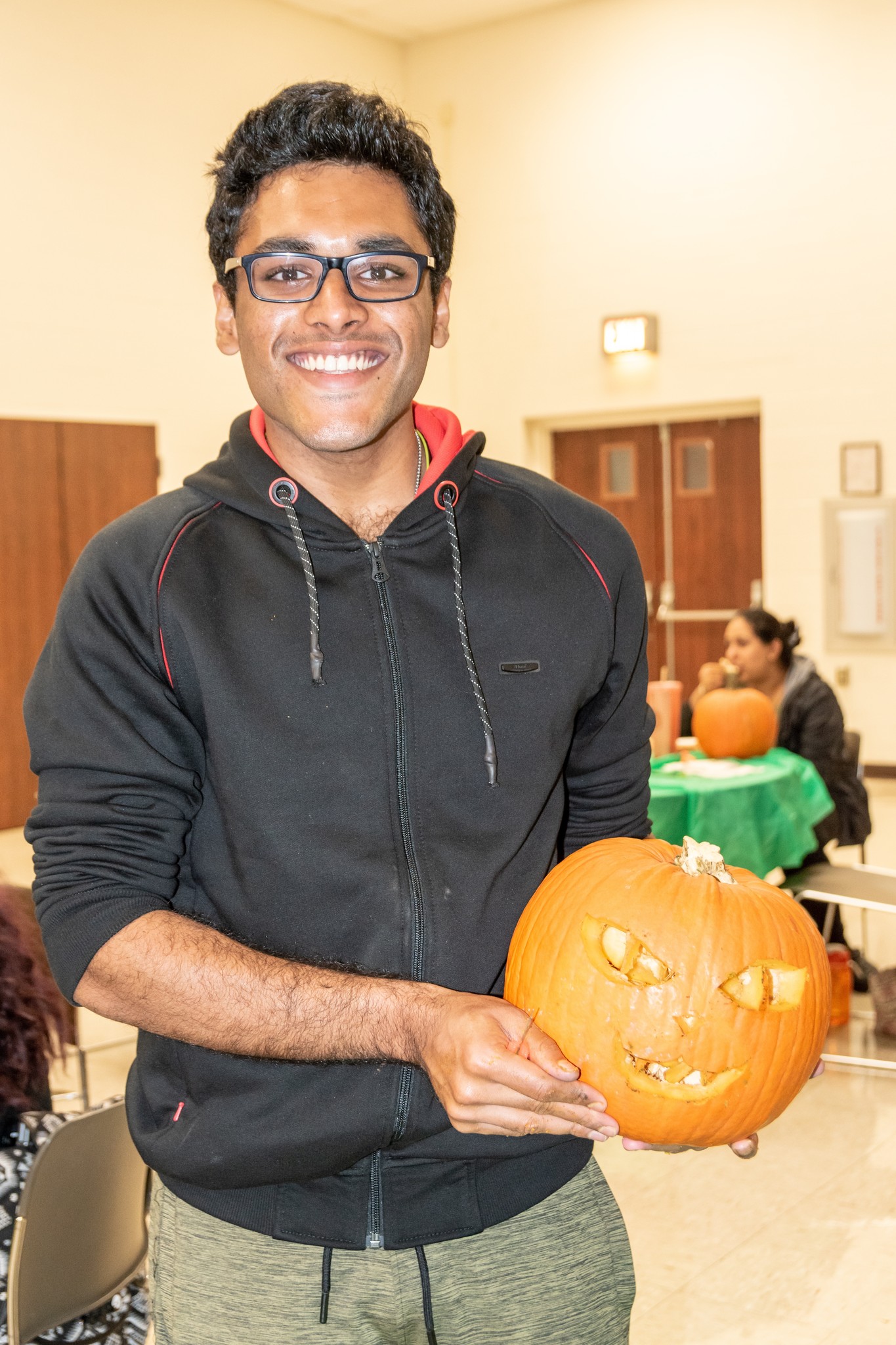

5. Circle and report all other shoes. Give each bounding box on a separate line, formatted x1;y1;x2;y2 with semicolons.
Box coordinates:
850;949;880;993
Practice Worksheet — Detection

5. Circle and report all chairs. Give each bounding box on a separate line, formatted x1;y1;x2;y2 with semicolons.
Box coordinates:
820;727;871;962
7;1093;157;1344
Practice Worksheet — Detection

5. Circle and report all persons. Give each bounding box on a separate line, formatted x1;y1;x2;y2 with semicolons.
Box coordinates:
680;606;874;993
24;79;826;1344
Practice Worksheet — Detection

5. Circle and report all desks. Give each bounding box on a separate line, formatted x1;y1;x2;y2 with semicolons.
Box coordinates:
648;729;830;893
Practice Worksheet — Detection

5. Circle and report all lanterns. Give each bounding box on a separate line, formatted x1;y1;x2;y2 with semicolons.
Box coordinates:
492;835;831;1144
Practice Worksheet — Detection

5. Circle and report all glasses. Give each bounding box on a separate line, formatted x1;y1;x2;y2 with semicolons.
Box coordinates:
224;251;438;303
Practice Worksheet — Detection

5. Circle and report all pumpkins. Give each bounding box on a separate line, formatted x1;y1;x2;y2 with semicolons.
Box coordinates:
691;666;778;760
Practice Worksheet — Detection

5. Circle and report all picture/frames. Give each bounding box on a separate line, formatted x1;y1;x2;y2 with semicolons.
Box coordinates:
840;441;883;500
